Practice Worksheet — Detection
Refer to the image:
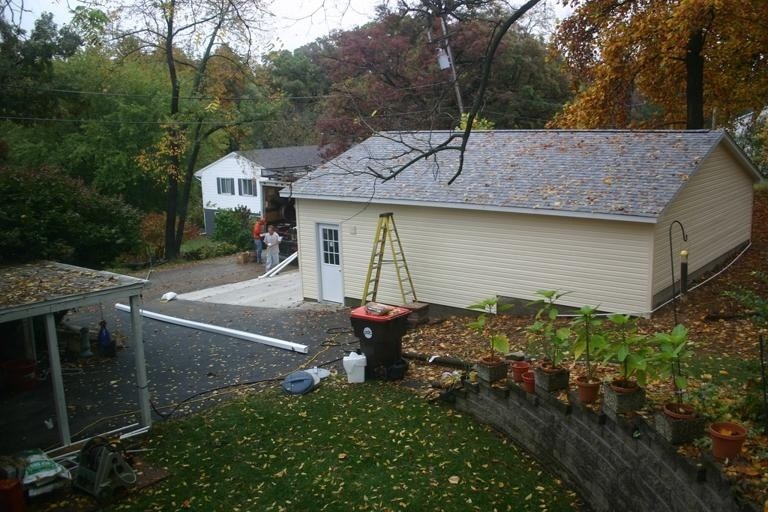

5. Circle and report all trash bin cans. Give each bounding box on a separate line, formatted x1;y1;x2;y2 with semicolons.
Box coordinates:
351;301;411;379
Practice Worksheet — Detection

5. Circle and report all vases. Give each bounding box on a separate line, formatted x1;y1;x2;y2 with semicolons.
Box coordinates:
708;421;746;461
521;372;534;394
511;360;529;382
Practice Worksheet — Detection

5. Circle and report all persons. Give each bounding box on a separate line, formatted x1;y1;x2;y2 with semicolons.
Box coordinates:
253;219;264;264
264;224;282;275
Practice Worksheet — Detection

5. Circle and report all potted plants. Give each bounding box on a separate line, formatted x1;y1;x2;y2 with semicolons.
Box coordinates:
465;298;515;382
525;290;708;445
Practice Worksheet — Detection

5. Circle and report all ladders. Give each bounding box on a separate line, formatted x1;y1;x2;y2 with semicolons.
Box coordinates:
360;212;418;306
